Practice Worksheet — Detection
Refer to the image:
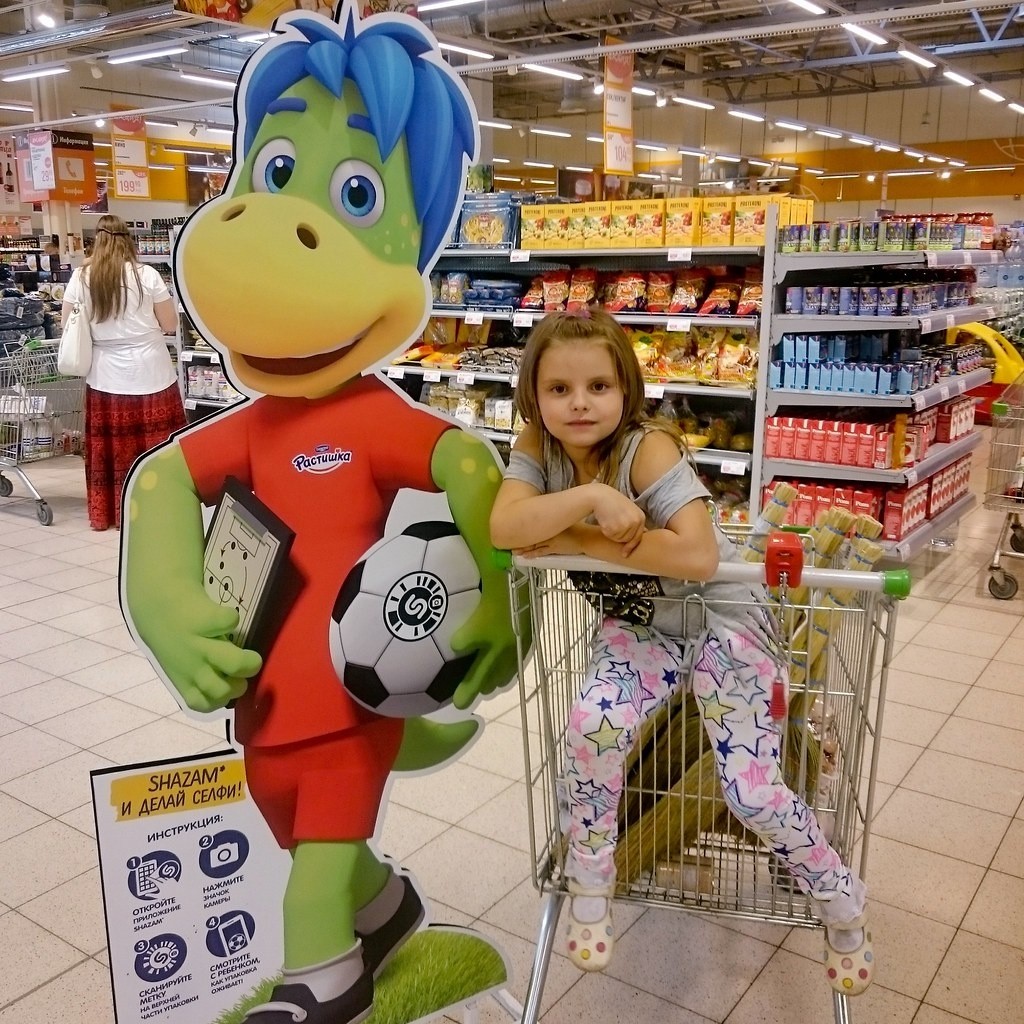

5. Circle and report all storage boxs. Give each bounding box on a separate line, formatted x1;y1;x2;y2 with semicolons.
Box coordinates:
765;393;975;470
168;225;992;568
485;395;517;430
521;196;814;250
768;331;985;396
765;453;973;542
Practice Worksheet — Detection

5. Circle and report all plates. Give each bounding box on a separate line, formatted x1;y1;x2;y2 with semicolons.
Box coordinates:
41;256;50;271
52;286;64;300
28;255;37;270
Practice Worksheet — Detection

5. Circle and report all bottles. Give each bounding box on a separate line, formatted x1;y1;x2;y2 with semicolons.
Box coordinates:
0;414;62;459
0;235;40;248
151;216;188;235
931;518;958;546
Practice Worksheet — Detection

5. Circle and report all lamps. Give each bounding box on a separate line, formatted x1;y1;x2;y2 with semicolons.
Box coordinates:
144;117;179;129
185;165;229;174
189;123;204;137
107;42;190;65
2;64;71;83
148;164;176;171
90;62;103;80
163;145;226;156
431;30;966;187
792;0;1024;120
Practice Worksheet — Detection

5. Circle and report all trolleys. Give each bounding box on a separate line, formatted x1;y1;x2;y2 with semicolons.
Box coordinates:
491;522;911;1024
983;371;1024;601
0;336;85;526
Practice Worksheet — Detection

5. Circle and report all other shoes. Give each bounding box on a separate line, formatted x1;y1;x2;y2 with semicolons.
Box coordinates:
824;926;876;996
566;878;613;971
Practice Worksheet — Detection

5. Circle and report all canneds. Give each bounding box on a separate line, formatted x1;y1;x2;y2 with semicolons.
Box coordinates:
786;282;969;318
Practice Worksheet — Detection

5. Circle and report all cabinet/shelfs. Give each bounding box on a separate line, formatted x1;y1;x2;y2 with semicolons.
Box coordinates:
0;225;176;356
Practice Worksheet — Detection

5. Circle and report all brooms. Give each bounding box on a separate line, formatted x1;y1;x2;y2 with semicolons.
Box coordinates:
531;479;884;899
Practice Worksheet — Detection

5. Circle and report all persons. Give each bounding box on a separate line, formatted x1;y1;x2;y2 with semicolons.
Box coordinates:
61;213;190;530
487;306;877;997
992;228;1011;250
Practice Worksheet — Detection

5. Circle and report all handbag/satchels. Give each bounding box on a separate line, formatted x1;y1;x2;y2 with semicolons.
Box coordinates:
58;268;92;376
